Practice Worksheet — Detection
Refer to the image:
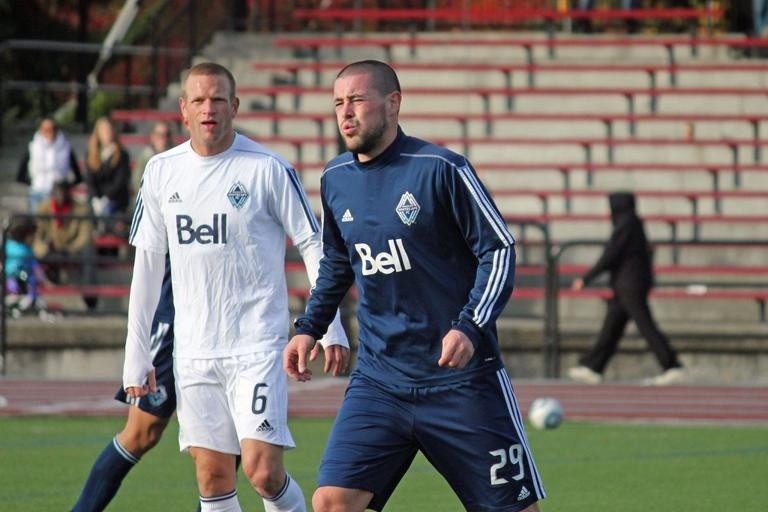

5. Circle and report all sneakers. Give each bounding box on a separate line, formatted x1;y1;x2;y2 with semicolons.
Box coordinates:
563;363;602;387
647;365;688;388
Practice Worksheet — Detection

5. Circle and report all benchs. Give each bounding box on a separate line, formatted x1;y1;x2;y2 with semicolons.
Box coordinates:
6;7;766;326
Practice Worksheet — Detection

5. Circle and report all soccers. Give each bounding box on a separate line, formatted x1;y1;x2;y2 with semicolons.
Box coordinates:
530;397;563;430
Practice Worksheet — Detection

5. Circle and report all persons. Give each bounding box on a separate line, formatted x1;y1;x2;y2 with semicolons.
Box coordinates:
1;113;177;322
566;188;688;388
123;62;351;510
281;60;546;512
70;254;242;511
